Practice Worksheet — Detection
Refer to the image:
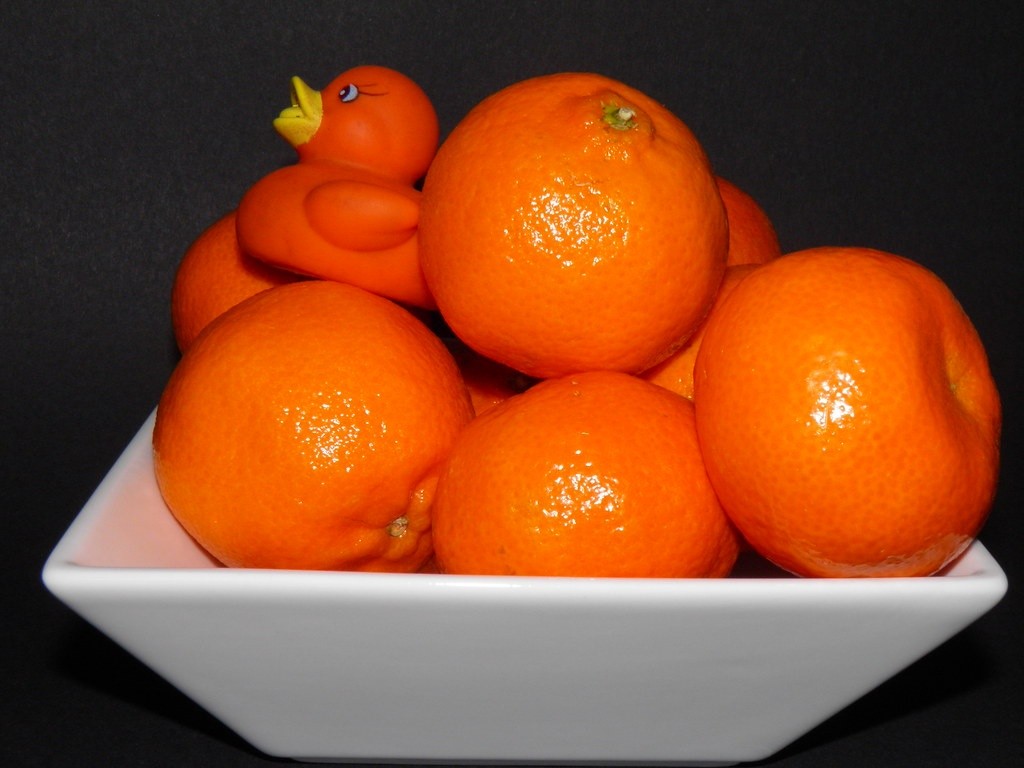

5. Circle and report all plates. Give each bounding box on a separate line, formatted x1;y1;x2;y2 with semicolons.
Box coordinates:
41;405;1007;763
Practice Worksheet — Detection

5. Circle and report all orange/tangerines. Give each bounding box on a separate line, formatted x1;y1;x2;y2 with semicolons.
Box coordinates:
153;73;1000;580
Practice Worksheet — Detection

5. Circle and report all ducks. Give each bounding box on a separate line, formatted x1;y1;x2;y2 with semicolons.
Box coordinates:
236;65;440;311
